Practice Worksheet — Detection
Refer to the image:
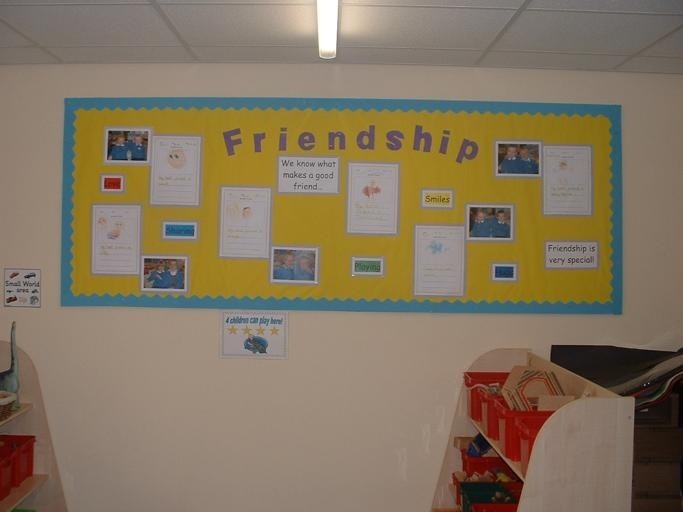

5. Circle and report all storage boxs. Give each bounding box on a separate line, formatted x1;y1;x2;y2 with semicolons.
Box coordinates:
0;433;36;485
448;369;553;512
0;450;15;500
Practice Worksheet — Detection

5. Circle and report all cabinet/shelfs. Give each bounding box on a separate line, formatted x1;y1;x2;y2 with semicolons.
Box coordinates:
430;347;635;512
551;345;681;512
0;339;68;511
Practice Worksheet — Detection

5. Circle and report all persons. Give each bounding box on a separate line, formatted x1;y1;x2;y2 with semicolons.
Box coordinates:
490;209;510;238
127;135;147;160
498;144;519;173
111;132;128;160
164;260;183;289
146;261;171;288
469;208;491;237
273;251;298;280
506;145;539;174
296;255;314;280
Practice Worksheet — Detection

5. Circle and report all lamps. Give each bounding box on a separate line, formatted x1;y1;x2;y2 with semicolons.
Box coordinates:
315;1;339;63
